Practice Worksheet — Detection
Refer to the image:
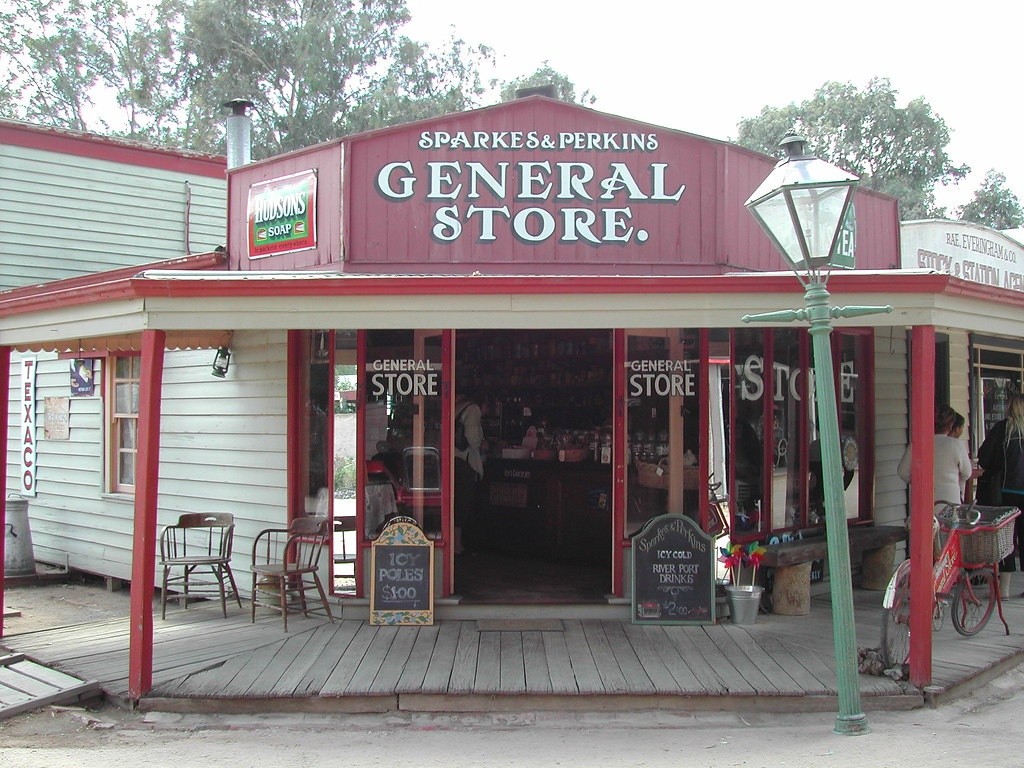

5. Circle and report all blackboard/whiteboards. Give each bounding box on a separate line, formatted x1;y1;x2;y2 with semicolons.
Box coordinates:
631;513;718;626
370;541;434;625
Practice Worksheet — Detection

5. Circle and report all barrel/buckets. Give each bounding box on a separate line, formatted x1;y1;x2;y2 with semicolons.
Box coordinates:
724;585;765;625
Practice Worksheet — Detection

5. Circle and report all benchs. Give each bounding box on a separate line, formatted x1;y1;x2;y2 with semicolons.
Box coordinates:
756;526;907;615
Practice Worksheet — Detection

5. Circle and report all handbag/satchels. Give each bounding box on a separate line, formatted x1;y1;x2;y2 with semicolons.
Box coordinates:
454;403;475;452
976;472;1002;506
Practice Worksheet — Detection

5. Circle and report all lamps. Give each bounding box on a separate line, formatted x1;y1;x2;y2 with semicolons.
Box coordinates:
211;348;231;379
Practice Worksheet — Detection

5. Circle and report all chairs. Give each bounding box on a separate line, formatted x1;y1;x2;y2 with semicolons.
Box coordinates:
396;445;441;534
159;512;242;619
250;517;335;632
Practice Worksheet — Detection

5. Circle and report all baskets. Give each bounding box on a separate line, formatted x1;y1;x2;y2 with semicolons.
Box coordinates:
934;503;1019;564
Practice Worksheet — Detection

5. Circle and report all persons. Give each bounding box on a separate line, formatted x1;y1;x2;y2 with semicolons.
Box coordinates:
948;410;985;503
978;391;1024;601
454;361;484;561
898;401;973;528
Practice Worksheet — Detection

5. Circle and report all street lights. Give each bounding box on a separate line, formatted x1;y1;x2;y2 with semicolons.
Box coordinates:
739;129;896;735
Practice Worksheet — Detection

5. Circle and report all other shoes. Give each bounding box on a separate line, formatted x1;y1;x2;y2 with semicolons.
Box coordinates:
983;593;1010;600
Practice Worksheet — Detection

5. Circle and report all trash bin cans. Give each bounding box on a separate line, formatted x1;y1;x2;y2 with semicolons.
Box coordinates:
4;493;36;574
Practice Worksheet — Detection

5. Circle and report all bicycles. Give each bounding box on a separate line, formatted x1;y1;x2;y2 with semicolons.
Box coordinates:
880;499;1021;670
